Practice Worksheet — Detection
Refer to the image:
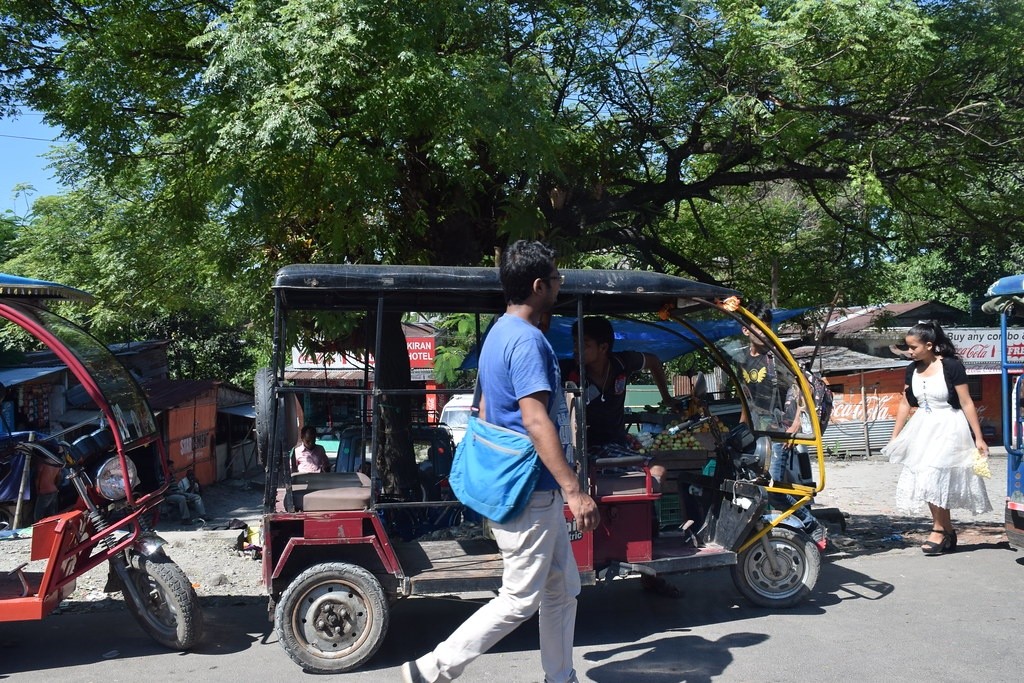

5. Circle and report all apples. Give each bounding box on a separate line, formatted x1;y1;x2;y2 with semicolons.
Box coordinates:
625;416;728;454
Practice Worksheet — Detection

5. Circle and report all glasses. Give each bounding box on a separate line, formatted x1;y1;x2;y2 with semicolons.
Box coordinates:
189;474;194;476
542;274;565;286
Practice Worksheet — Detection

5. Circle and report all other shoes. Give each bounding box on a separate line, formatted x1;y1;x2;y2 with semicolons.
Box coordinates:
182;518;194;525
198;514;212;522
402;660;430;683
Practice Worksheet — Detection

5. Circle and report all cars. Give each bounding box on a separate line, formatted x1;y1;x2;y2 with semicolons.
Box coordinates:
438;393;475;447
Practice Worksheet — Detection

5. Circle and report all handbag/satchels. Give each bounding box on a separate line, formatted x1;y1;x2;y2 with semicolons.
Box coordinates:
448;414;542;525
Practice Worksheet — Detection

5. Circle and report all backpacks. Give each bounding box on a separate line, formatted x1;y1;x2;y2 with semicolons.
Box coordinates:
784;368;833;437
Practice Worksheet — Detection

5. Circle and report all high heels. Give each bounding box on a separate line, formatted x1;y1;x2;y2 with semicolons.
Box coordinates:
921;529;951;553
950;529;957;547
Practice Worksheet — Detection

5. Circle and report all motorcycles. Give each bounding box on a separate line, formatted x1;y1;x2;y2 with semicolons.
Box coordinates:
255;262;834;677
1;273;203;651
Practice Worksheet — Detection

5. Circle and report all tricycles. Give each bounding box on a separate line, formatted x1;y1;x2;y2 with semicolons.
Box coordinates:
981;274;1024;551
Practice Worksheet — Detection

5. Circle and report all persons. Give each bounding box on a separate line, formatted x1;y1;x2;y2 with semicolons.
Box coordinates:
688;302;827;552
882;317;991;555
289;424;332;475
158;459;214;526
32;438;95;524
567;315;677;542
400;235;602;682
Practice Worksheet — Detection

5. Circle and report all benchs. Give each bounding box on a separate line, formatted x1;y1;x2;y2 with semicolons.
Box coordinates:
277;392;371;510
565;381;652;494
